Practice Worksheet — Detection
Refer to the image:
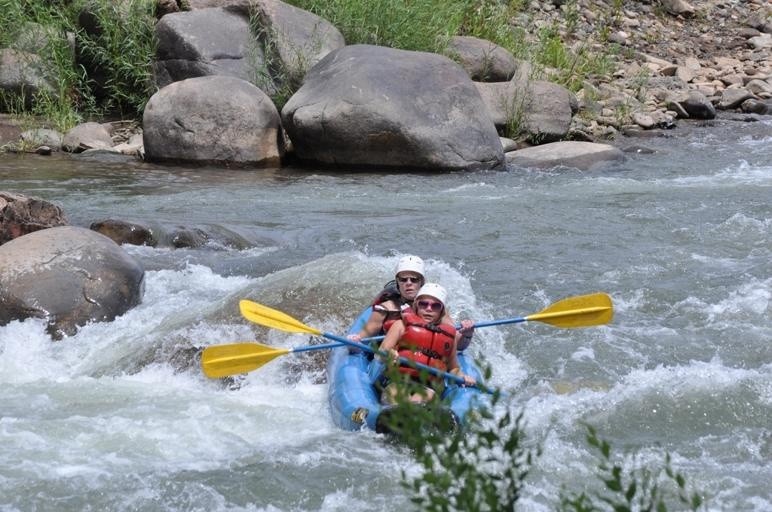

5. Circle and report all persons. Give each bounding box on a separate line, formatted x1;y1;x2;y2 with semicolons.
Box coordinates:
344;254;475;353
372;281;476;404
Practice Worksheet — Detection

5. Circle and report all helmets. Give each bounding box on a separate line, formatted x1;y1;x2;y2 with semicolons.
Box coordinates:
394;256;426;287
413;282;447;314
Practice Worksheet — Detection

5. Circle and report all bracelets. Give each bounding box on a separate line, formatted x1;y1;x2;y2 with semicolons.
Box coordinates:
463;334;473;340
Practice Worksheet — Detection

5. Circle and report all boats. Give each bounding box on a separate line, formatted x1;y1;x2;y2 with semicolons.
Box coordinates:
327;303;486;436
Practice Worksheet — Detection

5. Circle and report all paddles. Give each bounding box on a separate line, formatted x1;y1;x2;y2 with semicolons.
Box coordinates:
201;292;613;379
239;298;497;395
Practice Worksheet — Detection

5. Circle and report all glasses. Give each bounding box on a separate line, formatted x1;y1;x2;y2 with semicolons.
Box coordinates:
396;276;424;282
417;300;442;310
